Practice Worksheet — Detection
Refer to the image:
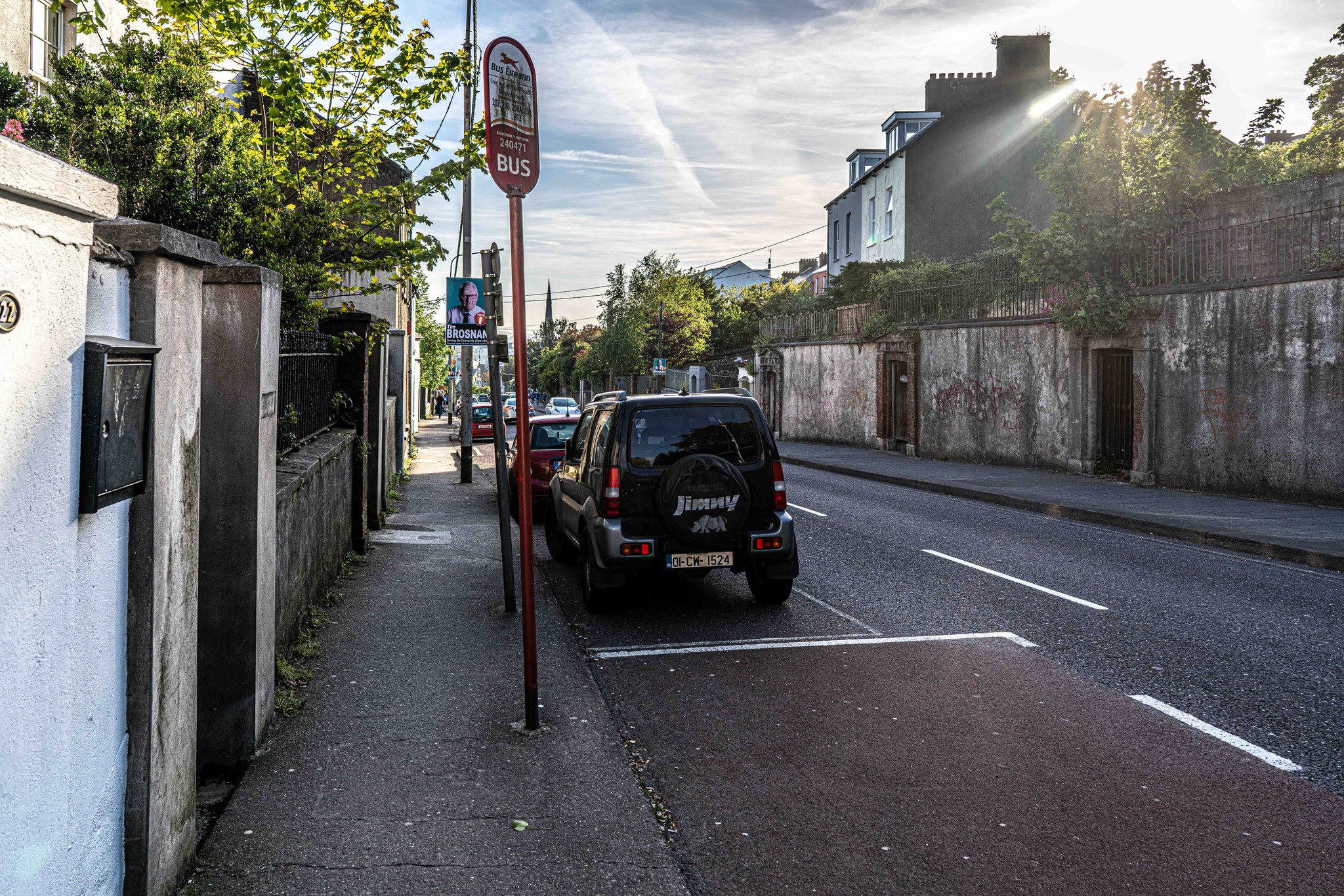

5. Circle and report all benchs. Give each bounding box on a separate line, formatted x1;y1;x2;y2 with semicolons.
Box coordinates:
535;437;567;446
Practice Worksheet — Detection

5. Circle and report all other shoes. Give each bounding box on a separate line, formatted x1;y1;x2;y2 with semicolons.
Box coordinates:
439;417;441;419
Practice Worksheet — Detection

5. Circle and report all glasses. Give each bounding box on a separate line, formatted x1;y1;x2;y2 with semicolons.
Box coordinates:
459;294;477;299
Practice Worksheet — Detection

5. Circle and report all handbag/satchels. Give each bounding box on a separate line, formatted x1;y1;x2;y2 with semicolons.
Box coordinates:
435;404;440;411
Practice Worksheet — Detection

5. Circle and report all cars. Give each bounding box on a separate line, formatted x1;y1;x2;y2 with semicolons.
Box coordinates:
505;413;610;525
545;397;582;415
501;398;536;423
528;392;537;399
456;403;506;442
455;392;516;421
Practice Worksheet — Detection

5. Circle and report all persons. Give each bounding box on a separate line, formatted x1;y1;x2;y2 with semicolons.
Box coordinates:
436;393;448;419
530;392;547;406
654;360;665;370
447;281;486;324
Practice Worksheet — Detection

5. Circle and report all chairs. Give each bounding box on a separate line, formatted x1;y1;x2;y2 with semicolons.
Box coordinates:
487;407;492;415
538;428;548;442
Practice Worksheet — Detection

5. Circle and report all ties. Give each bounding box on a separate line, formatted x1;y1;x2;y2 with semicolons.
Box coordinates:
462;313;470;324
658;367;659;369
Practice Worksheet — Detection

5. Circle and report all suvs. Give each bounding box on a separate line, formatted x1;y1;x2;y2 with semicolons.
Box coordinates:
543;386;799;616
